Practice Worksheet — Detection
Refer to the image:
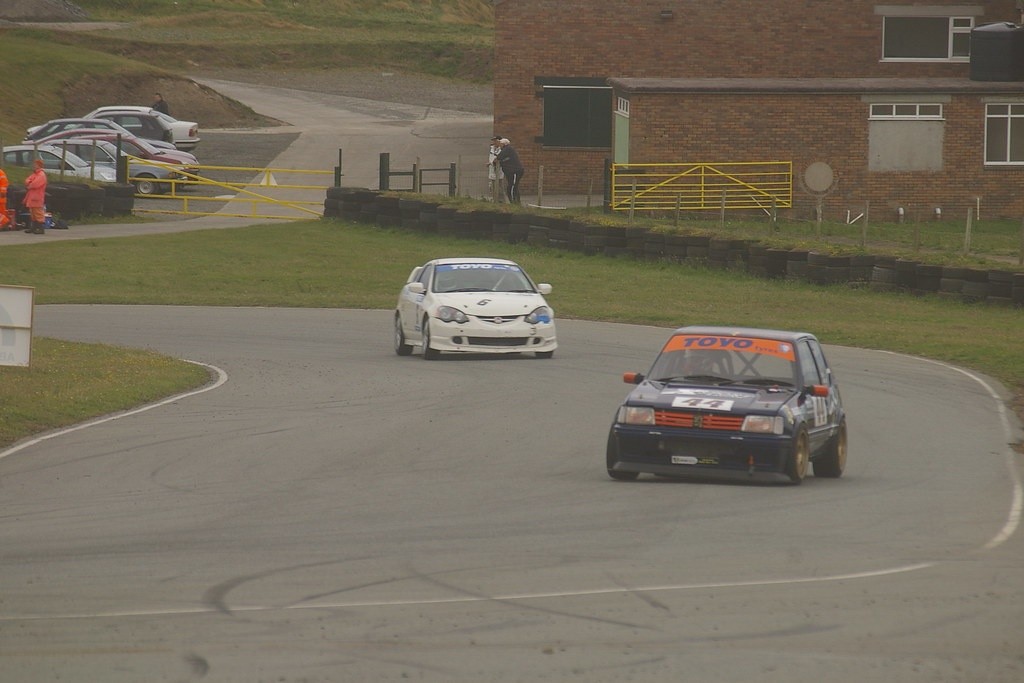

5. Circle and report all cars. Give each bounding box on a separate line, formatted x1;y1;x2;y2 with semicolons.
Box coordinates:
25;105;201;166
20;127;199;180
1;145;117;184
40;138;186;196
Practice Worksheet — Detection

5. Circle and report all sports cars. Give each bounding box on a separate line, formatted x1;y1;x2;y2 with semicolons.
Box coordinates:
605;326;848;485
393;256;557;359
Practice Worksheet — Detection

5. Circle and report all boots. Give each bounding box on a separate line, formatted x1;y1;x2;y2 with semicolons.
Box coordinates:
25;222;35;233
34;223;44;234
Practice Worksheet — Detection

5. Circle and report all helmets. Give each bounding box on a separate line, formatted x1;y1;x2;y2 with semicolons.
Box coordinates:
684;349;713;376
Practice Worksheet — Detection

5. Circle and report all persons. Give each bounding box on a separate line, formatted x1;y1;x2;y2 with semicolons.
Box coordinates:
22;160;48;234
152;93;169;116
438;272;458;291
681;349;713;374
493;138;524;203
488;136;504;203
0;168;9;228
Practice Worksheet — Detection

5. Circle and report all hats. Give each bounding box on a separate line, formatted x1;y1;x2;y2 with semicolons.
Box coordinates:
35;160;43;167
491;135;502;140
497;139;510;145
